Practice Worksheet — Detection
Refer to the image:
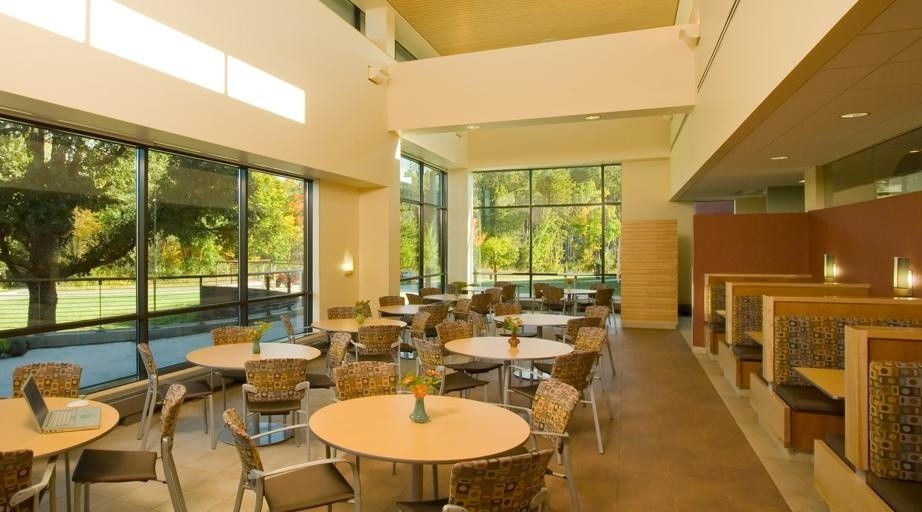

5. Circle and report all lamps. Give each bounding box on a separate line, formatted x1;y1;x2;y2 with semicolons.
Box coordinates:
823;254;837;284
344;255;354;277
892;256;913;298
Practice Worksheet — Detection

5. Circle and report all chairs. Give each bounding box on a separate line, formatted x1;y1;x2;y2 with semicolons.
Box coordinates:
137;342;217;452
13;362;82;399
72;383;188;512
0;449;58;512
